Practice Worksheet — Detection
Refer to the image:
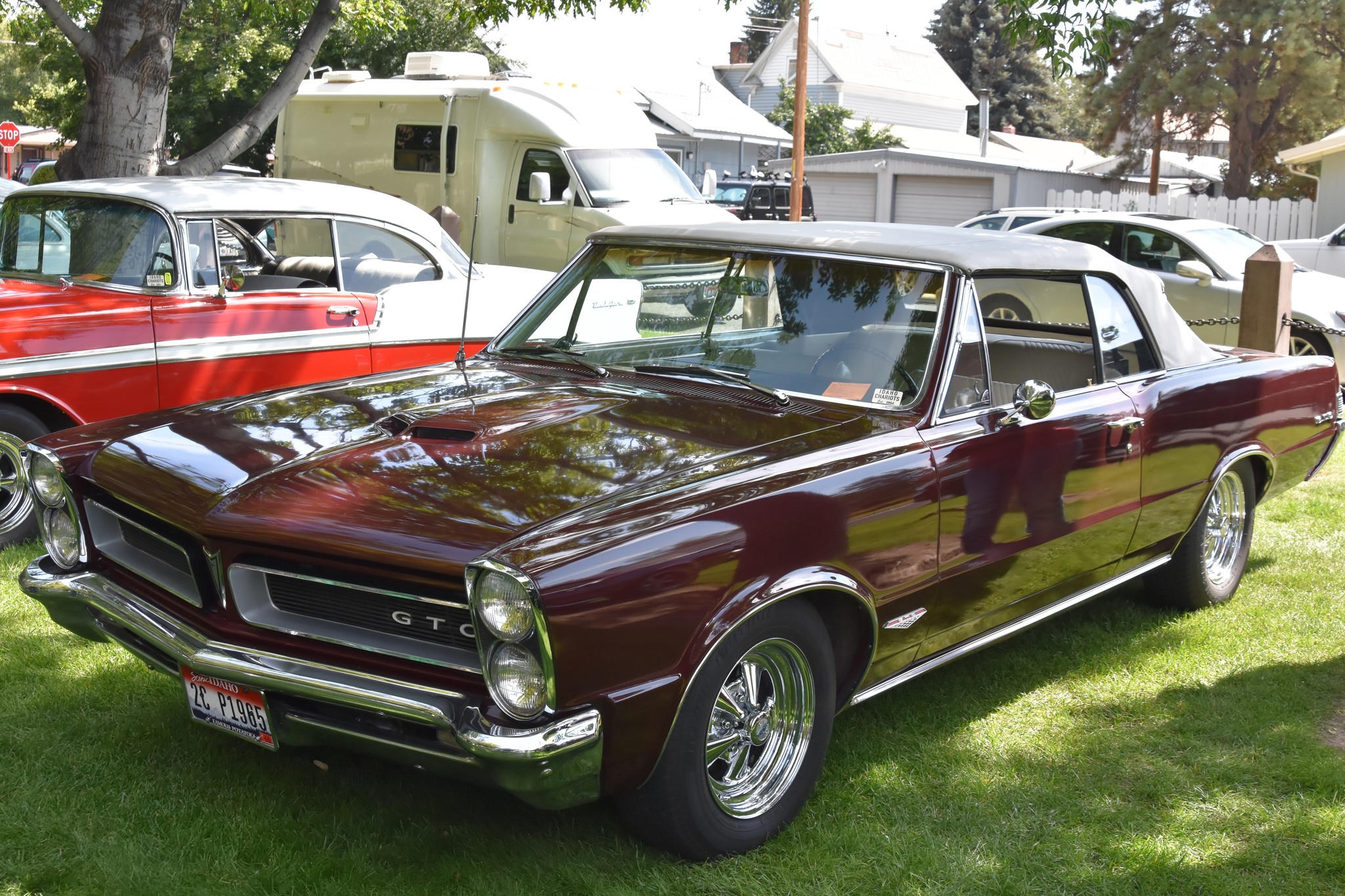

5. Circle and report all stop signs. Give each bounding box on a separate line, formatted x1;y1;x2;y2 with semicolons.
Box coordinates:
0;121;21;148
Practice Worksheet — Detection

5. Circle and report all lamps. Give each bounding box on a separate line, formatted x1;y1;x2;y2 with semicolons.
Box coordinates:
686;151;693;159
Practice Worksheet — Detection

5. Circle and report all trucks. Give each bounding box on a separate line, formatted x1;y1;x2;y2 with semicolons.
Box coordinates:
267;49;745;320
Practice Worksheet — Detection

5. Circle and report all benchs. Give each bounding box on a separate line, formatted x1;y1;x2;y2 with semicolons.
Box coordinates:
259;255;438;295
714;328;1092;411
152;269;329;290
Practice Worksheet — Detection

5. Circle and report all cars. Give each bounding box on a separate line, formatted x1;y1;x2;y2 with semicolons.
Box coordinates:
0;178;70;273
15;218;1342;864
0;174;646;554
945;205;1345;404
11;156;59;187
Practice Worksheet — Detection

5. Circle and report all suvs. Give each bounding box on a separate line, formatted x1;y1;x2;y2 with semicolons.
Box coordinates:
698;164;816;221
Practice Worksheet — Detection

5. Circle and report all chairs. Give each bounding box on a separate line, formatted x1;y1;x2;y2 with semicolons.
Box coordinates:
1128;235;1149;268
1148;235;1181;271
